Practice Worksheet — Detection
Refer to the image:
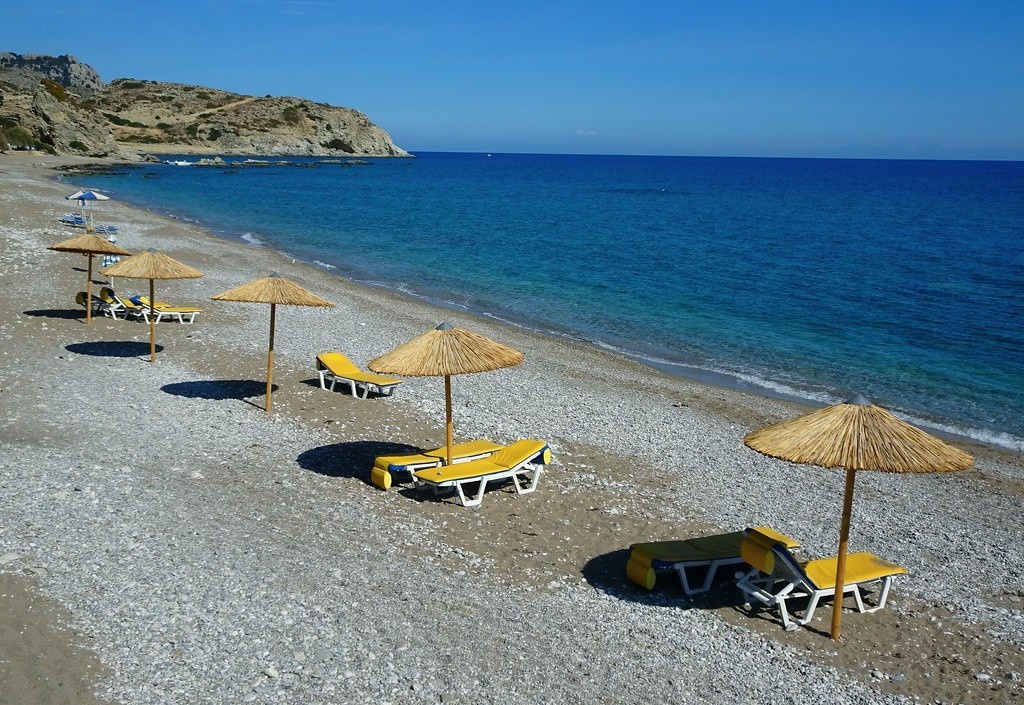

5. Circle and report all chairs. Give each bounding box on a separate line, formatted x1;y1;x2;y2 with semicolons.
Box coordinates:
370;441;506;492
61;211;118;238
316;353;403;401
733;527;907;630
413;440;551;507
626;527;804;599
75;286;203;325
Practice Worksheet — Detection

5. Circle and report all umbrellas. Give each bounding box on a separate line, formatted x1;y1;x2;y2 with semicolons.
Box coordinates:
65;187;110;230
97;247;205;362
744;394;977;640
101;234;121;287
211;271;336;412
367;321;525;465
47;228;133;325
